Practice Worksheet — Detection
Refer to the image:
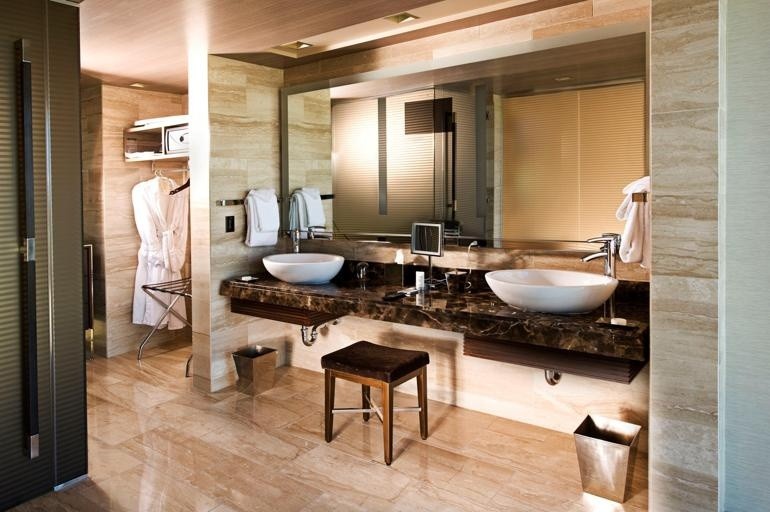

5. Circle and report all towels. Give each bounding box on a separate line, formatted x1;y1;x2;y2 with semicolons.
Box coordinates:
616;176;650;269
245;188;281;247
289;186;326;240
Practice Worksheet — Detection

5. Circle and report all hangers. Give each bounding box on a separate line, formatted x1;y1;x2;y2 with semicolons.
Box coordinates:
133;168;181;193
169;176;190;195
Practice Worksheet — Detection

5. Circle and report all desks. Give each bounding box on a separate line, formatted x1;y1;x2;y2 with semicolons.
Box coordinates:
136;276;191;378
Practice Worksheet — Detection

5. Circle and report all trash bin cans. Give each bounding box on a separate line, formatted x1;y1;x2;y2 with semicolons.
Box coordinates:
231;345;278;396
573;414;642;504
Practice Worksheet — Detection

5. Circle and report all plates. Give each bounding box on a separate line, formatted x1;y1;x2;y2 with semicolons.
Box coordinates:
235;278;259;282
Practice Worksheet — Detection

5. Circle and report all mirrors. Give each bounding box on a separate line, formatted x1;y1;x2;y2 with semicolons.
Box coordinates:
280;24;650;253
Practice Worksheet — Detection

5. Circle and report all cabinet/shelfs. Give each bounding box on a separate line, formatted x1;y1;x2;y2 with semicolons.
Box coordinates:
121;113;190;163
82;243;95;361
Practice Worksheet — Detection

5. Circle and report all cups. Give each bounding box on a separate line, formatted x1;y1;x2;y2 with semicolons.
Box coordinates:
242;276;252;280
356;262;369;280
445;271;467;295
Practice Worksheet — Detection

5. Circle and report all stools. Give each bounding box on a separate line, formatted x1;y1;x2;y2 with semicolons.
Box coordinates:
321;340;430;466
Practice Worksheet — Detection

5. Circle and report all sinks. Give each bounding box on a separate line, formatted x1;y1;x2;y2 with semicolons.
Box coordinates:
485;269;619;314
262;253;345;284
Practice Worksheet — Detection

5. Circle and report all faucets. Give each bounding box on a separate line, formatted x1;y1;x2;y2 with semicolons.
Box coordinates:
292;230;301;253
307;225;334;241
600;233;621;253
579;236;616;277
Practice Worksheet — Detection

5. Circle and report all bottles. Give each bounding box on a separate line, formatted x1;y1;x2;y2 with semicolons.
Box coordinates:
415;271;425;290
416;290;424;307
